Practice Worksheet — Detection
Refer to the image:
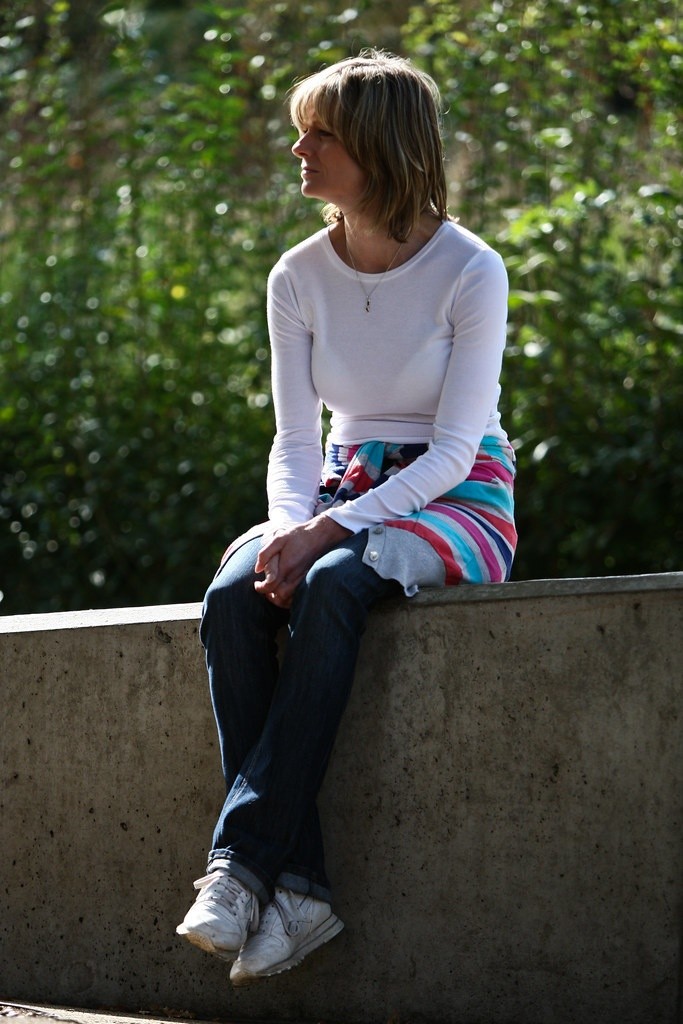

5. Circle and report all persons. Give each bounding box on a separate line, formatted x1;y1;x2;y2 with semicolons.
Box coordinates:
174;43;520;982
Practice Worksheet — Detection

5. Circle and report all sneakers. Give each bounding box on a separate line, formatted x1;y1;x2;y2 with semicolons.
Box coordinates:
176;869;260;962
229;886;345;986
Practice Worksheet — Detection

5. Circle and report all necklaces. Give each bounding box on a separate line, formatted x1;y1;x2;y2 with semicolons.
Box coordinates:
344;225;416;312
